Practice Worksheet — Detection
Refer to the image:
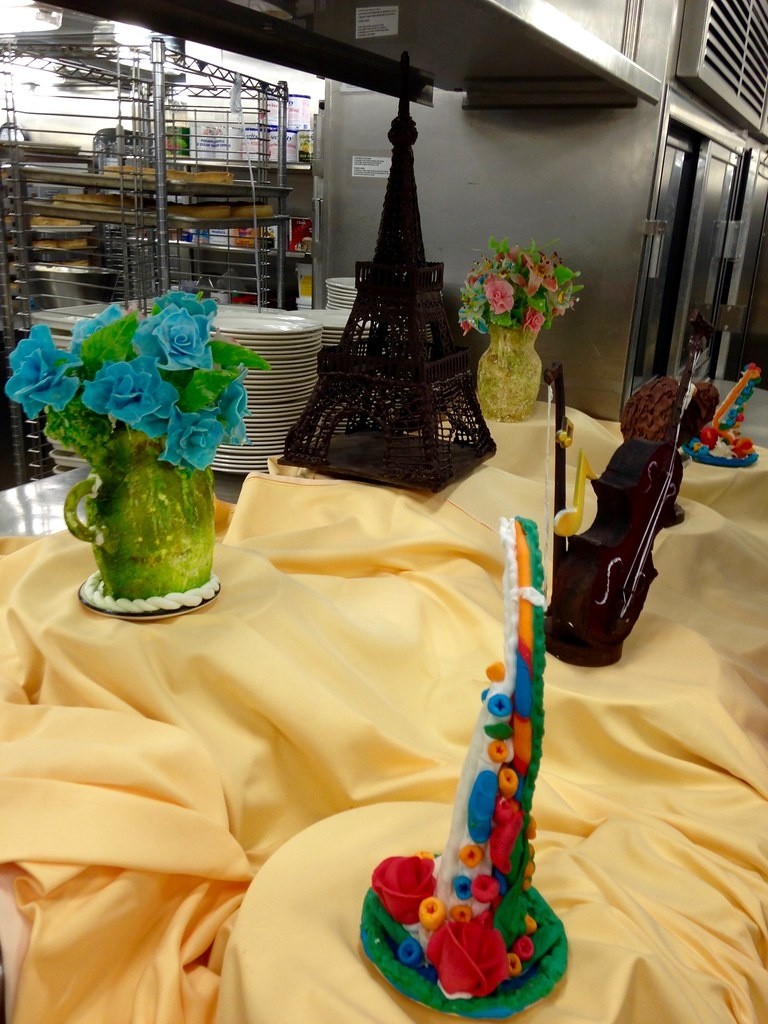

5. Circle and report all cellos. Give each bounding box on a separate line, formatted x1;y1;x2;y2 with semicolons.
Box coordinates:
550;307;717;652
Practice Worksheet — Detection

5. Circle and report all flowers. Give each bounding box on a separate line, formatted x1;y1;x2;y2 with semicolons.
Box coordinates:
5;291;272;474
457;235;587;337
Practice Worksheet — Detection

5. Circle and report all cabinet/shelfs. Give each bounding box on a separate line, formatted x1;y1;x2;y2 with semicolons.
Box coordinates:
0;34;311;491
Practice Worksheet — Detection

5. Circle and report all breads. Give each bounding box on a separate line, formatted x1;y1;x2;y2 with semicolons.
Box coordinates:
3;166;273;271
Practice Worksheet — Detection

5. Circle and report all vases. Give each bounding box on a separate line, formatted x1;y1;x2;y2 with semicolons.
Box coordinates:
477;323;543;423
64;429;216;600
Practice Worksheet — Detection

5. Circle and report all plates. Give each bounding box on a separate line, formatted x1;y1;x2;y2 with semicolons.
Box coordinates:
296;269;431;354
193;300;324;478
